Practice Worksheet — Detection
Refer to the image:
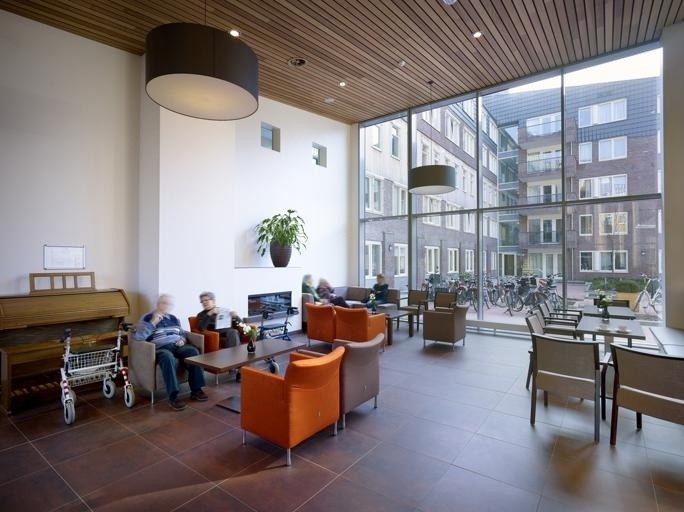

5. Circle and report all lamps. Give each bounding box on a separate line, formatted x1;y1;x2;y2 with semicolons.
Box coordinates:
137;0;260;123
405;80;456;197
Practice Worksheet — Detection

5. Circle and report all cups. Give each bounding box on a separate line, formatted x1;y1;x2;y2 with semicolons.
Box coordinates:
599;324;608;329
618;325;627;330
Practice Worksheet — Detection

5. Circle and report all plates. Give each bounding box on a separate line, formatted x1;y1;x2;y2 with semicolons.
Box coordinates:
615;329;631;333
597;328;611;331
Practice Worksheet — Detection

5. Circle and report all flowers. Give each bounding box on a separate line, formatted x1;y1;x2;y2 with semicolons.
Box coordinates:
593;288;606;303
231;318;258;340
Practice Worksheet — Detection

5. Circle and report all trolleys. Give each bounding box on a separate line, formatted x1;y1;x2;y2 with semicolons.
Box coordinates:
52;319;141;427
245;306;305;375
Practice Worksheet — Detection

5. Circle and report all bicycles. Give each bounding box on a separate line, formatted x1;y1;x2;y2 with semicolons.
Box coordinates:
633;272;664;314
423;265;563;318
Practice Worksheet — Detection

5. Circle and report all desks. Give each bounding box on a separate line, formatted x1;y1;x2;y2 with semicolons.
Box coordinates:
180;337;308;414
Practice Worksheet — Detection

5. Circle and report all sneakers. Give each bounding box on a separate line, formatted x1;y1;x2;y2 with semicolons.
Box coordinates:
190;390;208;401
167;397;186;411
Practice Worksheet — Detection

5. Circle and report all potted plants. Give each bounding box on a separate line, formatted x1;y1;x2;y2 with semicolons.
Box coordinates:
255;204;310;270
613;278;641;313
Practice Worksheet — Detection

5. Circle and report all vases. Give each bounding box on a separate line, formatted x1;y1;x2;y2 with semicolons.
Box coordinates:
246;335;255;355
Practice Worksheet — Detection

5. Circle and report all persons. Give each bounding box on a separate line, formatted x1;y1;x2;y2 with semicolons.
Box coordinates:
301;274;320;303
365;273;390;309
316;278;350;308
130;292;208;411
195;291;245;383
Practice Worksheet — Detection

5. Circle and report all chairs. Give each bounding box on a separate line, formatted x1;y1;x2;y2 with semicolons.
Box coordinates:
294;330;386;431
237;345;347;467
186;314;250;387
521;292;683;446
296;281;470;353
126;325;205;405
